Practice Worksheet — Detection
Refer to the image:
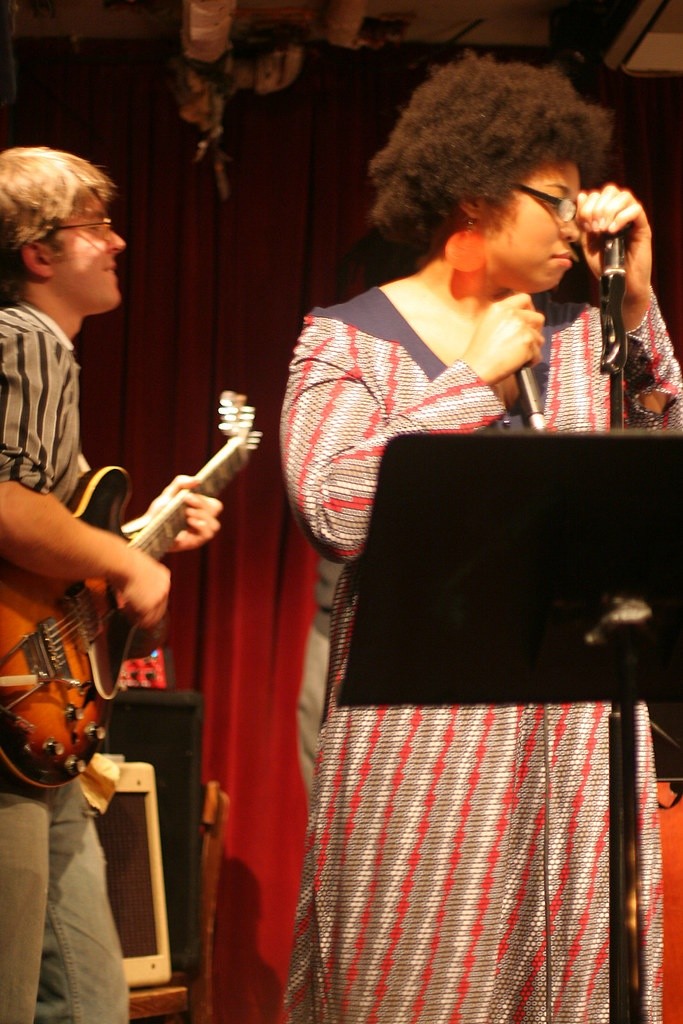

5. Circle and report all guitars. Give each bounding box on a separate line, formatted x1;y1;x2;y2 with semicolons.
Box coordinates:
0;389;266;794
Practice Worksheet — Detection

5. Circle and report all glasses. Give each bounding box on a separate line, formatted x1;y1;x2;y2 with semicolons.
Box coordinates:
510;183;577;223
44;217;112;241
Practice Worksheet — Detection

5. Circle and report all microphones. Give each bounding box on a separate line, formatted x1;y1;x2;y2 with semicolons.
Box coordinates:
516;365;546;432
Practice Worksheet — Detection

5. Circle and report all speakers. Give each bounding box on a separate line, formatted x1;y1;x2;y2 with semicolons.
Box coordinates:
106;689;203;970
89;761;171;988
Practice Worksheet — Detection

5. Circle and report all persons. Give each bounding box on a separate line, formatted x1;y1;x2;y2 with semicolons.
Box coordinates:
0;144;225;1024
280;50;683;1024
